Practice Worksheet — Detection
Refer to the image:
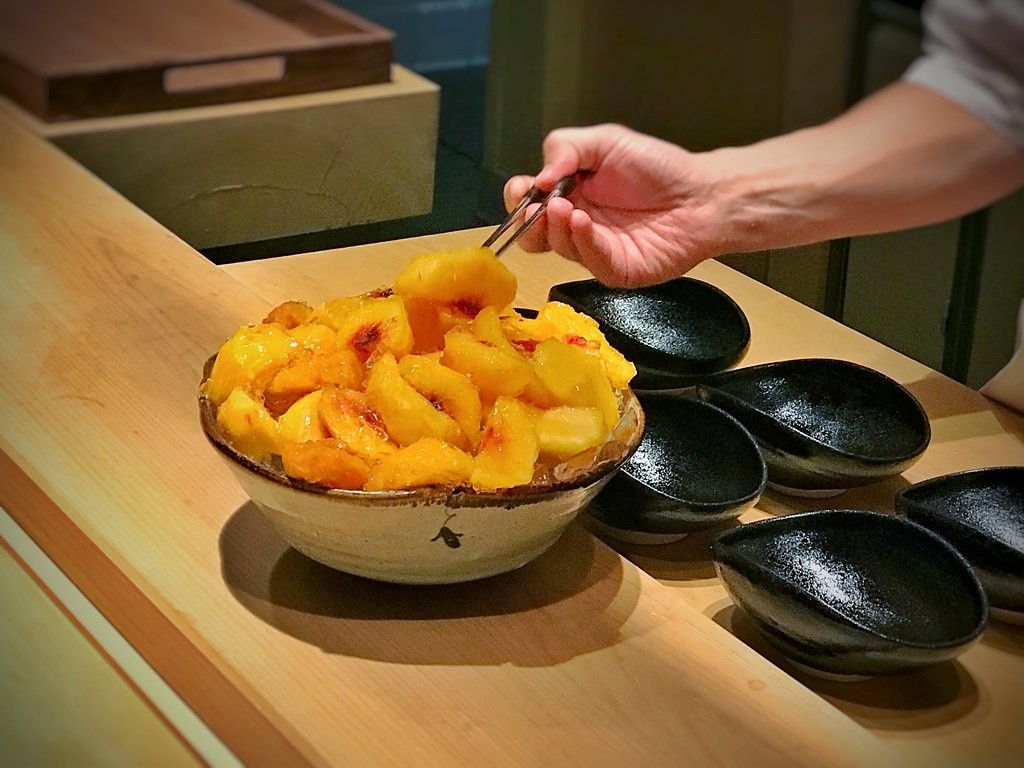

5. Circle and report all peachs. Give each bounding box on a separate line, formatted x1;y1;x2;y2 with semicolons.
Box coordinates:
211;247;637;489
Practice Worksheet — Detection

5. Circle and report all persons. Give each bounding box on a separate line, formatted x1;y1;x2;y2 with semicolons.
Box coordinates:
502;1;1024;411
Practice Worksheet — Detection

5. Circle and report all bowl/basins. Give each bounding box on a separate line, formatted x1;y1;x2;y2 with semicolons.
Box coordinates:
199;288;645;583
893;465;1024;627
695;358;931;501
548;276;752;396
586;389;767;544
708;510;988;683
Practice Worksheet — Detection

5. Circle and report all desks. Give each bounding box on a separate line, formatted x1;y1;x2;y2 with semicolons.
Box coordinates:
0;106;1024;766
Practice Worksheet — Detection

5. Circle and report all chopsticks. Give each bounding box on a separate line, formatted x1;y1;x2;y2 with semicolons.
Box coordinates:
480;176;569;257
